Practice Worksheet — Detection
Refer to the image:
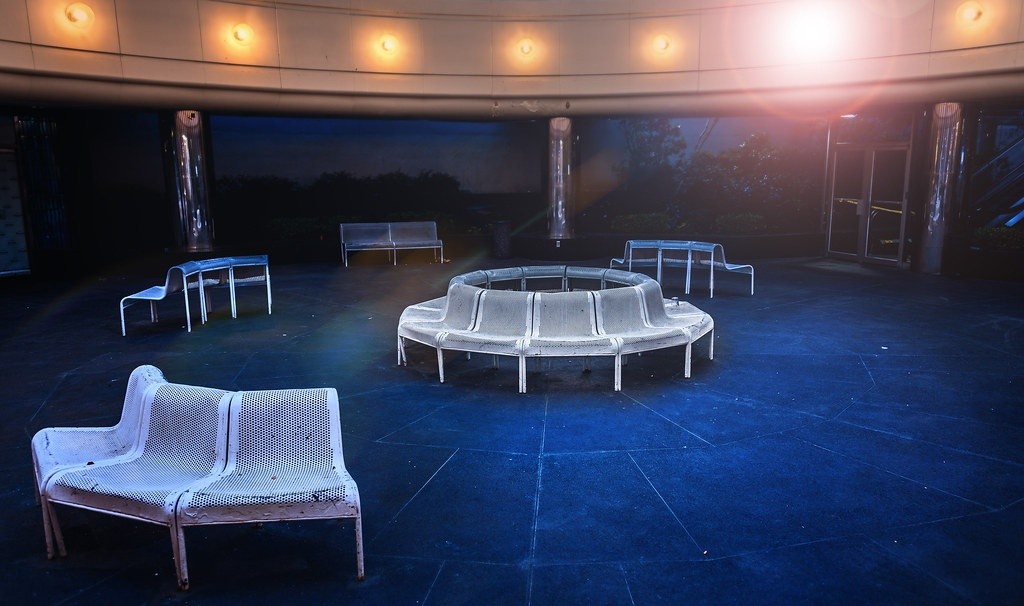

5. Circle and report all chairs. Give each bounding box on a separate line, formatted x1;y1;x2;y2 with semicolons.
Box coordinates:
591;286;689;392
43;382;235;591
396;277;465;366
603;239;754;306
175;388;365;590
208;255;272;318
636;280;714;377
438;289;535;393
414;270;488;308
632;272;711;358
521;265;567;292
120;261;205;336
566;266;607;292
153;257;235;321
485;267;524;291
339;221;444;266
31;365;166;559
398;283;485;382
522;292;619;393
